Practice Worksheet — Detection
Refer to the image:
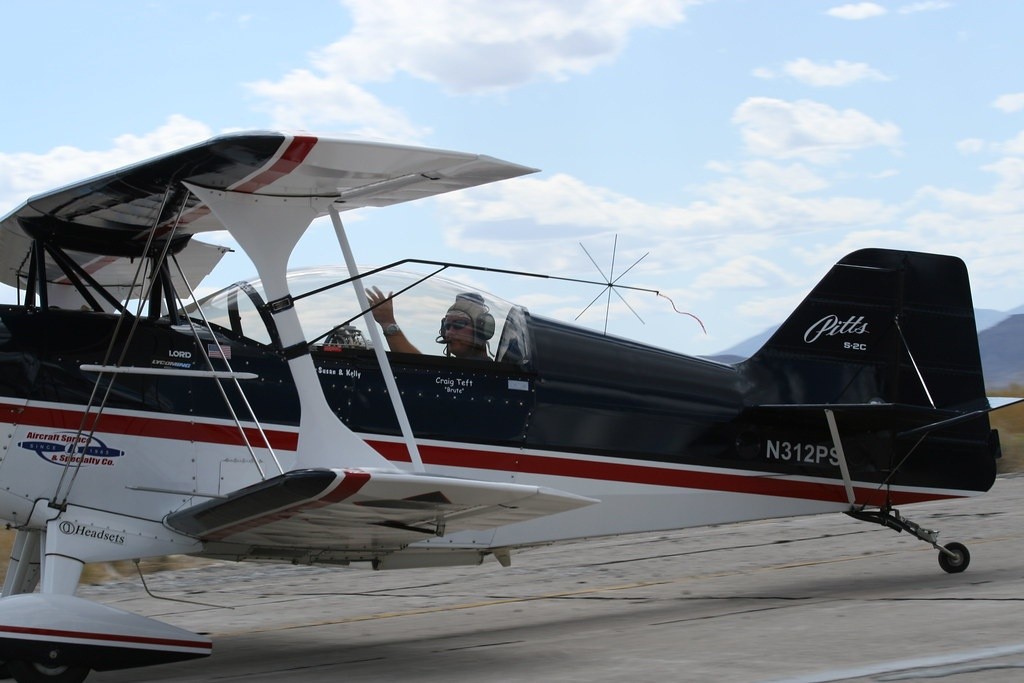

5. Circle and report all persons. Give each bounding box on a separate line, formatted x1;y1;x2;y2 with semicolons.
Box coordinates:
365;285;496;361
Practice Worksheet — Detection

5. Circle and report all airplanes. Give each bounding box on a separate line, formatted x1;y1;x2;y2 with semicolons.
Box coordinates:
0;129;1024;683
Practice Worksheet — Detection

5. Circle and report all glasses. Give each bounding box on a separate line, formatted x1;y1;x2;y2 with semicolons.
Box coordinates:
445;320;473;330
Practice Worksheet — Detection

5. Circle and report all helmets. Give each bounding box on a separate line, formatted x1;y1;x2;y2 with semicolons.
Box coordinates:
441;292;495;349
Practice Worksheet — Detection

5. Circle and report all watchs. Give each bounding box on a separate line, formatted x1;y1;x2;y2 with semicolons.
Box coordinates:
383;325;400;336
